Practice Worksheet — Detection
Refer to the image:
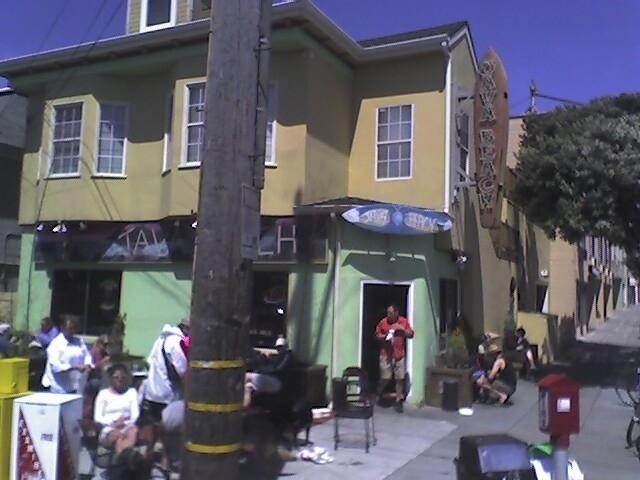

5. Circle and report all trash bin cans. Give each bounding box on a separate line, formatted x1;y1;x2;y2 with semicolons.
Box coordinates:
453;434;538;480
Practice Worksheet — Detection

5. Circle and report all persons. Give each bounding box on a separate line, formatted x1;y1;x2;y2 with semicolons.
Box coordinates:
514;325;537;370
33;315;60;350
138;316;192;474
93;362;143;465
243;335;293;411
0;322;19;358
37;309;97;398
373;304;417;414
89;334;112;375
474;342;517;408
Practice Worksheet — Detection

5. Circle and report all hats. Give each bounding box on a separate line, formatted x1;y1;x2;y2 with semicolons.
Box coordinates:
487;343;504;356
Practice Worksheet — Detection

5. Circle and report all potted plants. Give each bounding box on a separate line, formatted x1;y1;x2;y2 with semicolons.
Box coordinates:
441;322;475;406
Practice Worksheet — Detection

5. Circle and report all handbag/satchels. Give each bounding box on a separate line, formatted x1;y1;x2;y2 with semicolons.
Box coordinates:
162;358;187;383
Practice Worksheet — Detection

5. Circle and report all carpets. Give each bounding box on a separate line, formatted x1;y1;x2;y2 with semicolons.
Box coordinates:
276;409;459;480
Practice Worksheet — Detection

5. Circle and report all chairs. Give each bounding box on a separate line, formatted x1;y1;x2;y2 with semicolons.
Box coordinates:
80;364;377;480
